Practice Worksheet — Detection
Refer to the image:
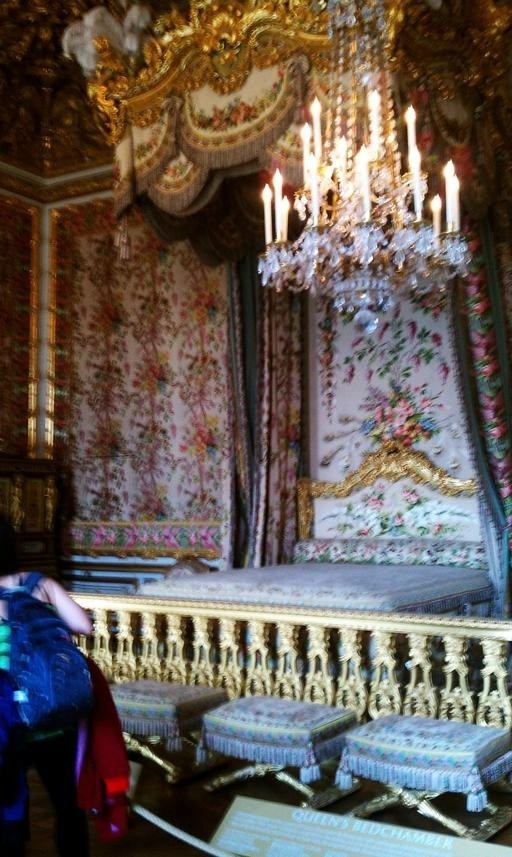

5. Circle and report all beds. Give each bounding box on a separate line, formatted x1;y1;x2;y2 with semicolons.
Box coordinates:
133;440;493;617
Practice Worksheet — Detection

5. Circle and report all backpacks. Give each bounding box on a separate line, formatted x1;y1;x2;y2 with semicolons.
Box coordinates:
0;571;96;732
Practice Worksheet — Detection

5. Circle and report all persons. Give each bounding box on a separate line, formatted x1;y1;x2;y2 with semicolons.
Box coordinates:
0;510;98;856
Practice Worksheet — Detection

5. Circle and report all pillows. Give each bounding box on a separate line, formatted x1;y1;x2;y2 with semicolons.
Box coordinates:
292;539;488;572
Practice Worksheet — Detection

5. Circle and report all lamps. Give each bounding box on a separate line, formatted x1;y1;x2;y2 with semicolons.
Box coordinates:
250;0;468;337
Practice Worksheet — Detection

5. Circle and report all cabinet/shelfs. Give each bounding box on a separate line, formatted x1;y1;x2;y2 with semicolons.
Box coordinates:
0;455;64;580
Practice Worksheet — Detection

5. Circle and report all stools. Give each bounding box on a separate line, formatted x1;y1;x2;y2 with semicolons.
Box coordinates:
335;711;512;843
202;693;362;811
110;679;228;784
